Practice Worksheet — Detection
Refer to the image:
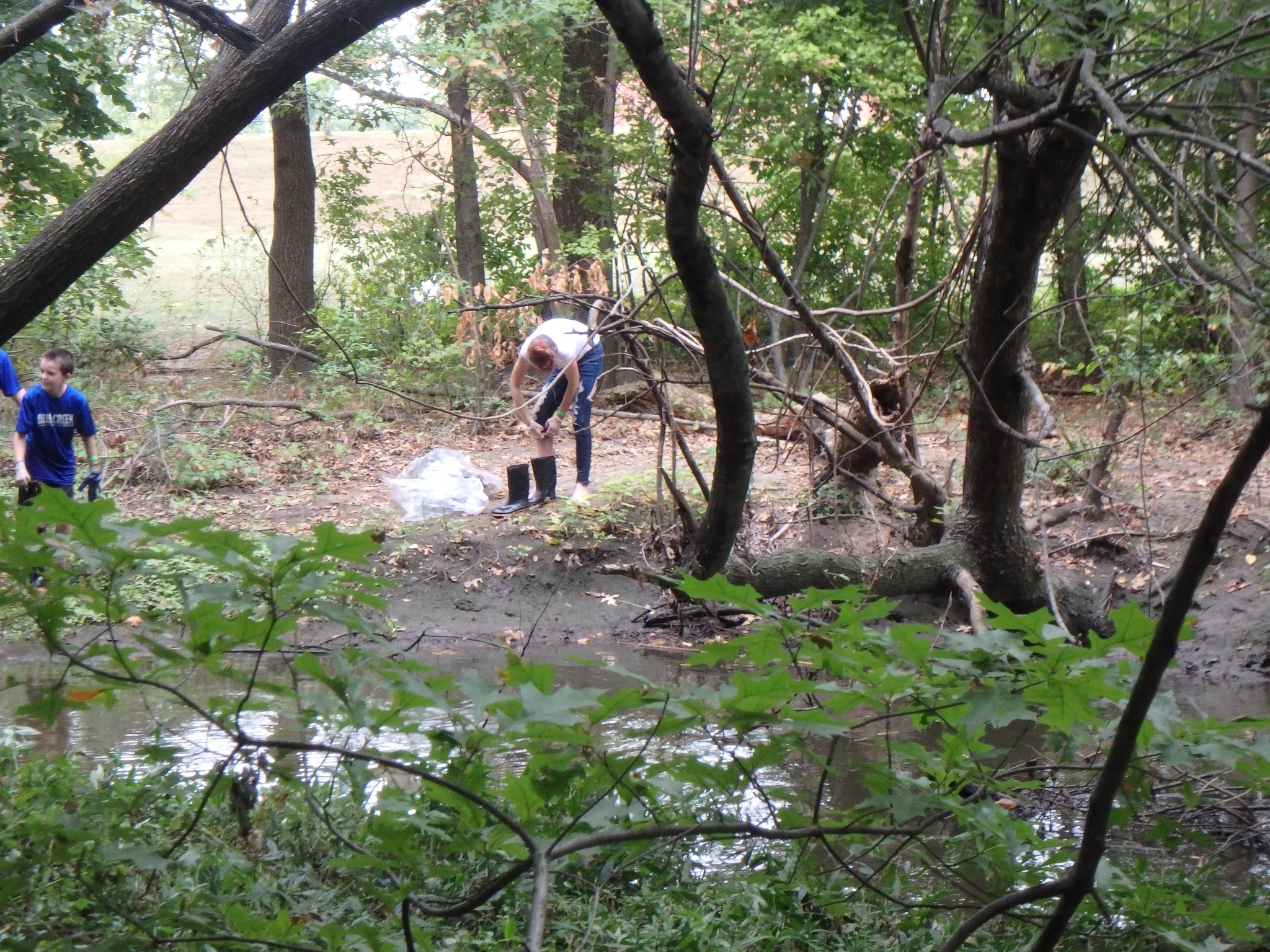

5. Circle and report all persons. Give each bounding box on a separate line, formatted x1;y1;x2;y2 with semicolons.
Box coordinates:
10;347;104;591
0;348;27;407
510;316;605;505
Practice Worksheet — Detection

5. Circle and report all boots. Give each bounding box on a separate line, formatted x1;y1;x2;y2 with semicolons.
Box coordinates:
491;463;530;517
529;456;557;508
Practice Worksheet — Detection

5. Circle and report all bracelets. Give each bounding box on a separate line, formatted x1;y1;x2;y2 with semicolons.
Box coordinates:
87;456;98;461
13;460;25;468
556;410;566;417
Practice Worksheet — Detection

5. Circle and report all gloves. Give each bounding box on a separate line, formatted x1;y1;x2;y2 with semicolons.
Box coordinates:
78;472;101;503
17;480;42;507
